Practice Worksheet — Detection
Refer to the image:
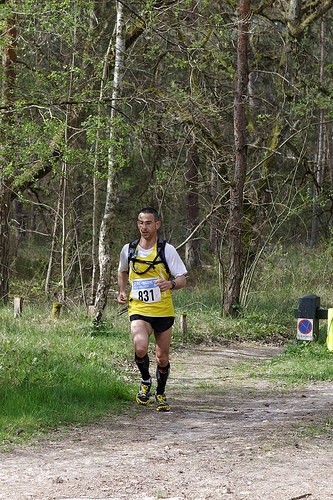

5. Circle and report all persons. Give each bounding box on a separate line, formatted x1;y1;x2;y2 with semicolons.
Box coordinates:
117;208;188;412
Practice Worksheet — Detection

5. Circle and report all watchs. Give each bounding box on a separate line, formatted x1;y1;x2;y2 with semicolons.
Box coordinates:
171;280;176;290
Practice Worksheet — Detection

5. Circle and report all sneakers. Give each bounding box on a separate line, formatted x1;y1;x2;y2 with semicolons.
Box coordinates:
136;383;150;405
154;392;171;412
